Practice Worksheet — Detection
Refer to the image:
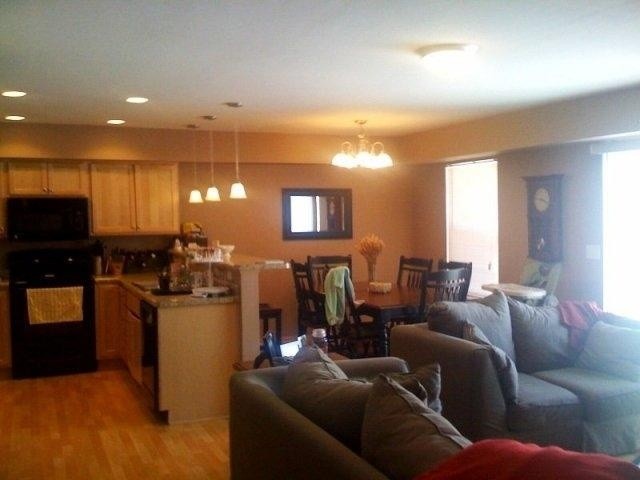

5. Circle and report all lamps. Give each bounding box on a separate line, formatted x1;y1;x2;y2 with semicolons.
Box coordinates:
332;121;394;169
186;124;203;204
201;115;220;201
227;102;248;199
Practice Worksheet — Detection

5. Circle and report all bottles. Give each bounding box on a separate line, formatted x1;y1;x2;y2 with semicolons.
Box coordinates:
311;329;329;357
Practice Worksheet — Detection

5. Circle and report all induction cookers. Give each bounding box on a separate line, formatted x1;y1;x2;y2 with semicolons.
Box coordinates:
12;248;92;283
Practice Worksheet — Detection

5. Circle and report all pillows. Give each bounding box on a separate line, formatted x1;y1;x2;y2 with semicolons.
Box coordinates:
463;319;519;406
508;292;585;374
427;288;517;369
575;319;640;381
360;371;475;479
281;327;442;449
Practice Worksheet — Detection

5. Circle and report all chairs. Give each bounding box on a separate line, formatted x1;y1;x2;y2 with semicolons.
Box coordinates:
289;254;473;359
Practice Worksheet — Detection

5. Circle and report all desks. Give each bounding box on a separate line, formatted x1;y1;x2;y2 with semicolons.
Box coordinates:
231;352;353;371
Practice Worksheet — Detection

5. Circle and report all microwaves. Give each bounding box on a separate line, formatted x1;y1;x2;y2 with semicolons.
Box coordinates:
9;197;90;242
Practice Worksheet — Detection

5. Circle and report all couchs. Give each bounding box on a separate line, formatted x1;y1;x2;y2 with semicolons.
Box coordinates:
228;356;640;479
388;308;640;456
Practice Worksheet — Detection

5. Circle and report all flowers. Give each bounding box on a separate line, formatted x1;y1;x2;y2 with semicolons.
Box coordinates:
354;233;385;266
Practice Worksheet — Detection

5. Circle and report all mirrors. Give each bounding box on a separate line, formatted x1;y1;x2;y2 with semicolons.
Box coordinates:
282;188;353;240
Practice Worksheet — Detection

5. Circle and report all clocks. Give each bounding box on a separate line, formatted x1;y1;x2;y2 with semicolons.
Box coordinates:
522;175;566;262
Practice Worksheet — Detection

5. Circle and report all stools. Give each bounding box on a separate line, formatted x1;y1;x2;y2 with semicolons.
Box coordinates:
254;308;282;368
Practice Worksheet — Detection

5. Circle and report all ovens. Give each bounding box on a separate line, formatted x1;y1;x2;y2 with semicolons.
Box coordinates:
13;282;97;377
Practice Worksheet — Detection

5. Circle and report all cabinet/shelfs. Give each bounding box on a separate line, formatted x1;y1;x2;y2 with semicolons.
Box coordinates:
0;157;90;197
142;304;240;425
0;285;14;370
97;283;120;361
91;161;181;237
119;282;143;389
